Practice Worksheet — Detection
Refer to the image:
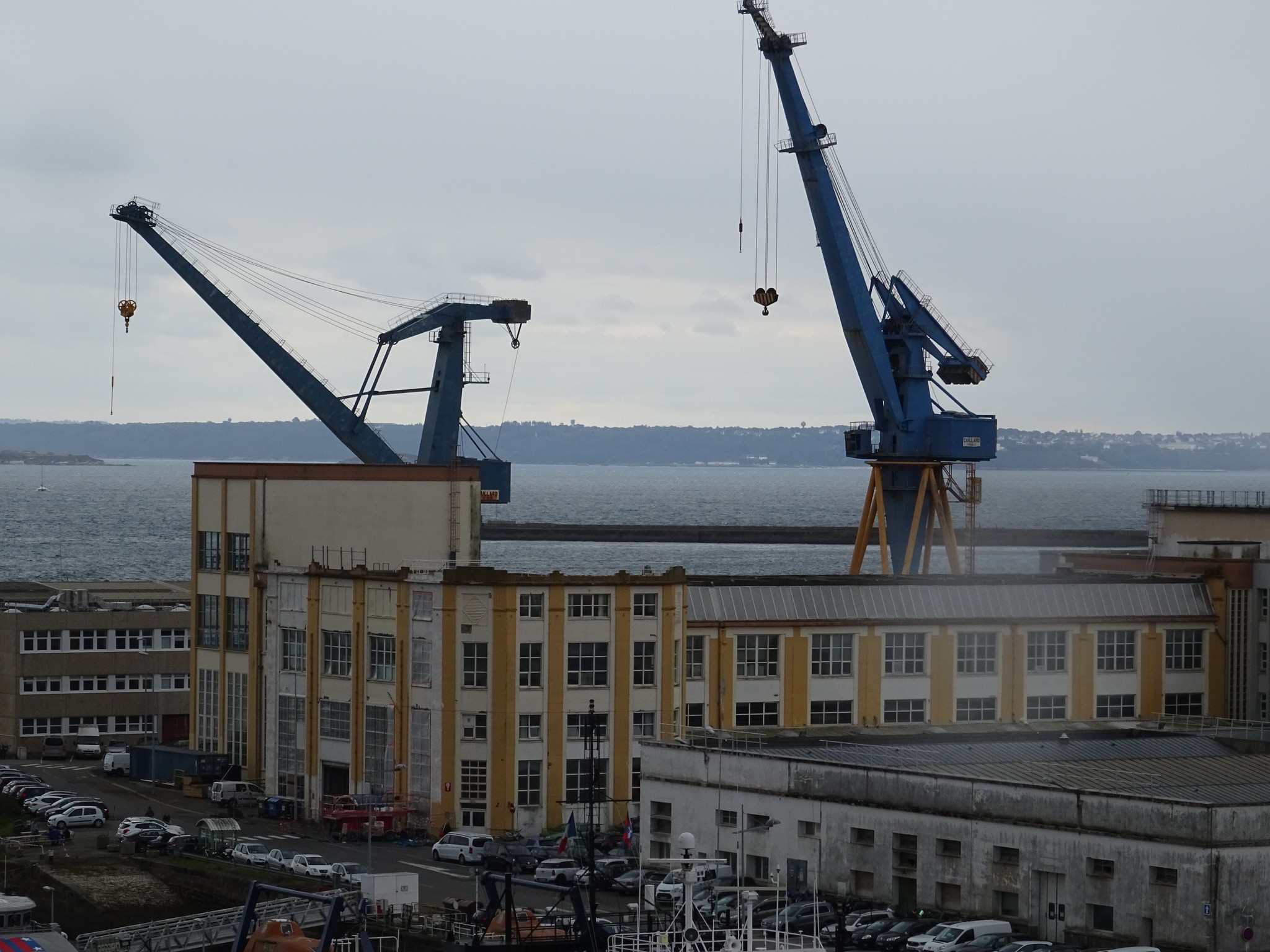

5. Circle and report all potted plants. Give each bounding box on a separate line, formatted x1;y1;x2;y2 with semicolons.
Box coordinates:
97;833;110;849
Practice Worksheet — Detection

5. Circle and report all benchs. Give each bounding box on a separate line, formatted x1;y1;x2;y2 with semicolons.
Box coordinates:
4;838;66;845
20;830;47;835
13;819;25;830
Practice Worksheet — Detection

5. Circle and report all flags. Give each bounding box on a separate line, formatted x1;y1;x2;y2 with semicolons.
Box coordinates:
557;809;578;856
621;810;633;854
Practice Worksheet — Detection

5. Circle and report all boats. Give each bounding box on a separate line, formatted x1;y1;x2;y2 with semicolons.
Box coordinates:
0;855;79;952
442;830;1161;952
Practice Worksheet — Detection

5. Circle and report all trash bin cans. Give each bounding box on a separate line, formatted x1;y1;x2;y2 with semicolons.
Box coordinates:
18;747;27;760
267;797;283;817
280;801;301;819
257;796;272;817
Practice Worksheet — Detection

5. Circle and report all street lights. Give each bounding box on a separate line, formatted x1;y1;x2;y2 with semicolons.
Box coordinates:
139;651;156;796
42;886;55;923
137;637;147;745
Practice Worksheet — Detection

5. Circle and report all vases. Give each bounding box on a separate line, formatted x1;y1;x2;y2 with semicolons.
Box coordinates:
123;841;135;855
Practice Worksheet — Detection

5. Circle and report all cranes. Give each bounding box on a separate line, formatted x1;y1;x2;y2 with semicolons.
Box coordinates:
734;0;999;576
106;192;534;505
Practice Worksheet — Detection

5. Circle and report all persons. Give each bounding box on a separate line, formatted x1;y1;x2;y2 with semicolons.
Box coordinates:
163;811;172;826
49;826;71;845
145;806;155;818
31;820;38;842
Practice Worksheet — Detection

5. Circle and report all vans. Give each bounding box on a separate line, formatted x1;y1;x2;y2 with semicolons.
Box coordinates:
42;736;67;761
103;752;130;778
431;831;494;866
73;724;104;760
211;781;266;808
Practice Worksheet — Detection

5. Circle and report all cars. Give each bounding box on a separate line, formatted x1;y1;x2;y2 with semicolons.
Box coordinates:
230;842;271;867
264;848;303;873
104;739;130;755
0;764;237;860
290;853;332;879
325;861;368;887
